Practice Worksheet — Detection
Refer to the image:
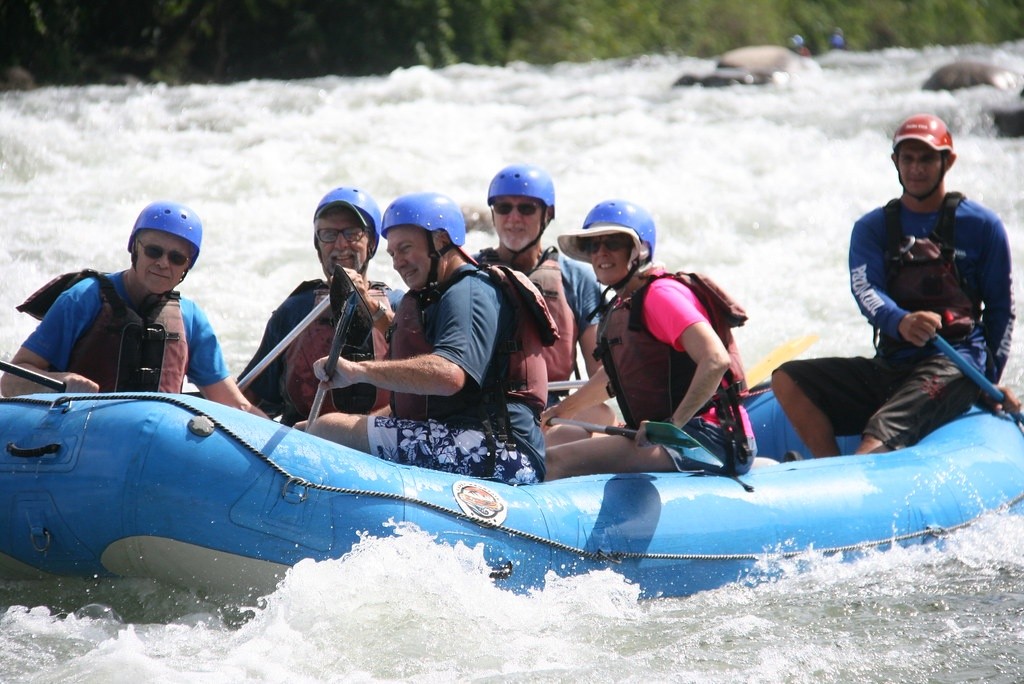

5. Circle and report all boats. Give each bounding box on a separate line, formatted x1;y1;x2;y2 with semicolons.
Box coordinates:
0;389;1024;629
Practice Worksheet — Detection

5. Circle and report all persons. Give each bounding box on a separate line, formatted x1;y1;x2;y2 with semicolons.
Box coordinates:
771;113;1021;464
470;164;617;426
540;199;758;479
0;202;269;418
294;192;558;486
235;188;404;425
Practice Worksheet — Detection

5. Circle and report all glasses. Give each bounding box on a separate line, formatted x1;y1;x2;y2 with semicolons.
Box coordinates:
493;200;537;216
586;238;627;253
134;236;193;265
316;226;373;244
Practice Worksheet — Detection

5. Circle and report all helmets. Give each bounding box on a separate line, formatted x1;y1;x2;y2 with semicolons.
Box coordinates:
381;192;465;246
893;115;953;153
558;200;654;271
487;165;554;206
128;201;202;269
314;187;382;245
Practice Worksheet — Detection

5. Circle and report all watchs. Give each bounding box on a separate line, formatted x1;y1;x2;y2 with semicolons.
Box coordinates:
373;302;387;320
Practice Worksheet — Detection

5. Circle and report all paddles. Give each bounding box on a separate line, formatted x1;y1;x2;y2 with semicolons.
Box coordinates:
547;379;593;390
929;330;1024;425
546;416;703;450
306;290;357;433
236;293;334;391
1;361;68;390
